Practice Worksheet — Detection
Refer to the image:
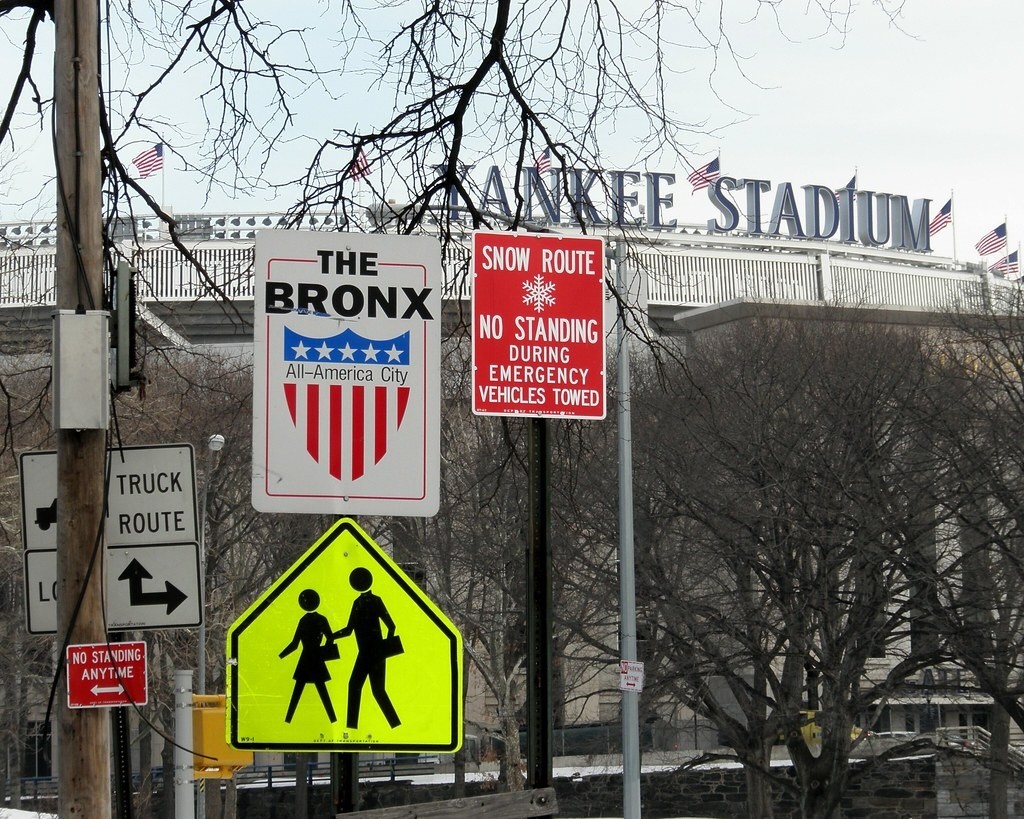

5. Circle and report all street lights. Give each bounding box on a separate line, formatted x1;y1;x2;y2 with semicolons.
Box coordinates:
367;203;644;819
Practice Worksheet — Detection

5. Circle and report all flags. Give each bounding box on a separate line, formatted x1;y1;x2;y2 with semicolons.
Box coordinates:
930;198;951;238
989;249;1018;275
974;223;1006;256
350;139;373;181
687;156;722;196
834;176;857;210
133;140;163;181
534;145;551;180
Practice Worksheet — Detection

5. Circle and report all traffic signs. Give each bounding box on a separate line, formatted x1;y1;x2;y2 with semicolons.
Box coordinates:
18;443;202;633
